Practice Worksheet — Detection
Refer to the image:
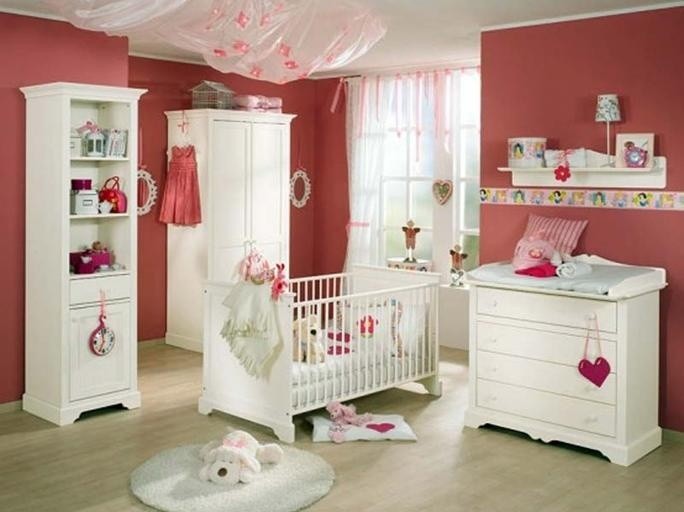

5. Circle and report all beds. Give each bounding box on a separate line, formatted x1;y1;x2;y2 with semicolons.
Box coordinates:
197;265;439;442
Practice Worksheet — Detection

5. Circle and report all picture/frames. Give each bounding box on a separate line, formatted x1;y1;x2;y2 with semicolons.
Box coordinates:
615;132;655;168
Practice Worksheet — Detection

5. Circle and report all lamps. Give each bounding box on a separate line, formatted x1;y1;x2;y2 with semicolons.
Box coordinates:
594;91;623;167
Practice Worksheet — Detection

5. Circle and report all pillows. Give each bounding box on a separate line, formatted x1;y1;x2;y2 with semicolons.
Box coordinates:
341;304;397;354
523;212;589;260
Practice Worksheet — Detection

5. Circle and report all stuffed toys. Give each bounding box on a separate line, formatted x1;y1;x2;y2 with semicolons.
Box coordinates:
92;240;104;253
323;399;376;444
292;313;327;364
270;262;290;303
196;430;283;487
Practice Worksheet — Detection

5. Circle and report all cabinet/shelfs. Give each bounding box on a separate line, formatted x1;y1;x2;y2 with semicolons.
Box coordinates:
16;79;148;426
164;109;294;355
465;261;667;467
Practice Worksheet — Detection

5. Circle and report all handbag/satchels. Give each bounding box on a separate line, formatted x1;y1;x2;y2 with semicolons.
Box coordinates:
98;175;129;214
578;308;611;390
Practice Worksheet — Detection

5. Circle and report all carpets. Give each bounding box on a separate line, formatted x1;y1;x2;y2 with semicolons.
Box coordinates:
128;439;334;511
